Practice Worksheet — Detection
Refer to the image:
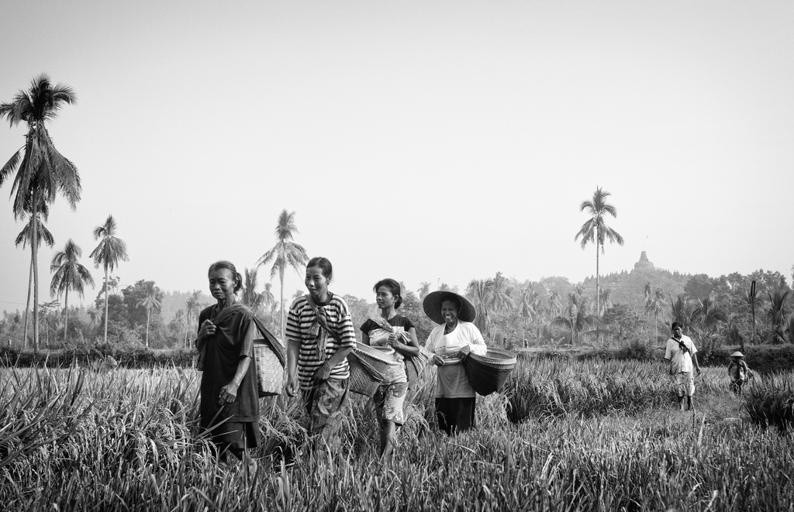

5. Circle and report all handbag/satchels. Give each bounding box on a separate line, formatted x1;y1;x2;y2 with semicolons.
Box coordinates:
404;344;434;383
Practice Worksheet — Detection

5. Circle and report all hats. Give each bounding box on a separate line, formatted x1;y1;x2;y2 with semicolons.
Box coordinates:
422;287;477;324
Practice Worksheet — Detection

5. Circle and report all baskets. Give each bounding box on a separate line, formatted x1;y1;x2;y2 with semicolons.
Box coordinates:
252;336;288;397
347;340;402;400
462;342;517;396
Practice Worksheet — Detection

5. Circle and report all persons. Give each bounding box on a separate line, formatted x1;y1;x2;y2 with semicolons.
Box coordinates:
194;260;260;457
359;278;420;456
664;320;702;412
285;256;357;455
420;290;488;436
727;351;749;398
